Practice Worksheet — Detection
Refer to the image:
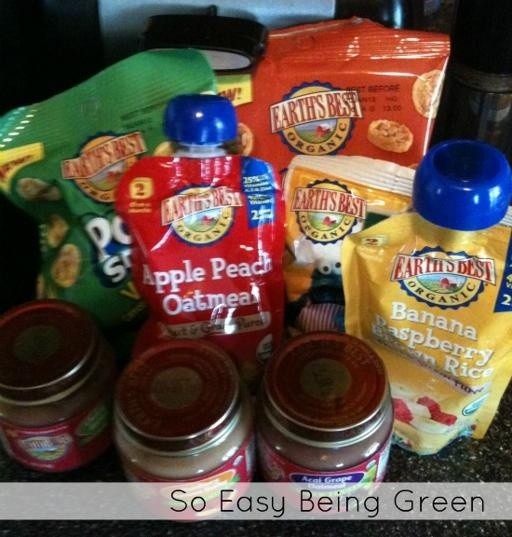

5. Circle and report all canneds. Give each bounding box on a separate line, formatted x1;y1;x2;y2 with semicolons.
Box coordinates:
255;330;395;515
112;338;256;523
0;298;118;472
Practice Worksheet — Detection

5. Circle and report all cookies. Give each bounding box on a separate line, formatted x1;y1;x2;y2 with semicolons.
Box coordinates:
367;119;414;153
411;69;445;120
237;122;254;158
